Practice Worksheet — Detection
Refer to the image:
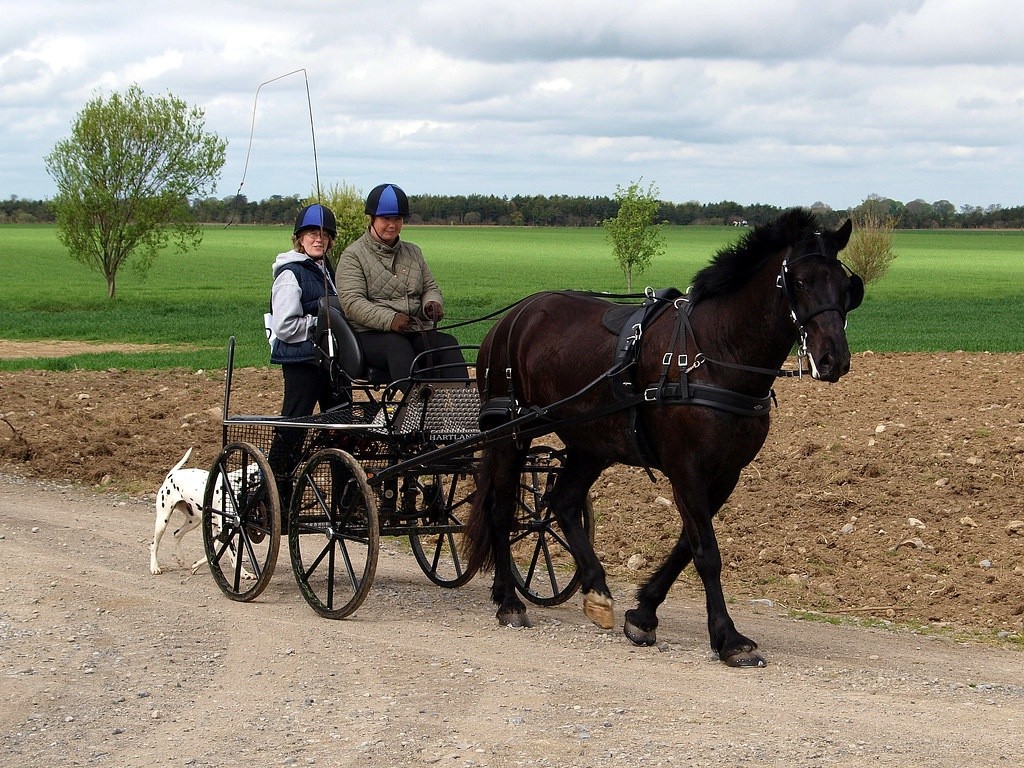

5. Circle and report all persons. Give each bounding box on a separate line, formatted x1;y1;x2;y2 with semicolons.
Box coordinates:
335;185;468;380
259;204;359;516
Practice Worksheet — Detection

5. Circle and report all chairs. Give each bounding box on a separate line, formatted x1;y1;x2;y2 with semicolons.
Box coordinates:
310;293;423;406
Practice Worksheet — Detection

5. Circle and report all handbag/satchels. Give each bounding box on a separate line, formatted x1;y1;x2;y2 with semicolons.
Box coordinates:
269;337;321;364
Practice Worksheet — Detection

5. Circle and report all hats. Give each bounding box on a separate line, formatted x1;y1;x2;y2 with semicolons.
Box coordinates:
294;204;338;237
365;184;410;215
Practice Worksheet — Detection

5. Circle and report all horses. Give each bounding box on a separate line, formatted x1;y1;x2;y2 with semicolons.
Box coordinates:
456;205;854;669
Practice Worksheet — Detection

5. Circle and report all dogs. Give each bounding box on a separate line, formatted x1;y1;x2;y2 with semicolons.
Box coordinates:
149;447;268;580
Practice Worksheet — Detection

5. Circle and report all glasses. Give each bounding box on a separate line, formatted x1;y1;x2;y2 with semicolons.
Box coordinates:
302;231;329;240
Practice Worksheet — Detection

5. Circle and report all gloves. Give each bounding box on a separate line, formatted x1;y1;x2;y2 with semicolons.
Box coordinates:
390;313;412;332
424;301;444;322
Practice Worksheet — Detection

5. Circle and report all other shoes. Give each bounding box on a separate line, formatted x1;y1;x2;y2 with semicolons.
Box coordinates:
329;491;348;518
